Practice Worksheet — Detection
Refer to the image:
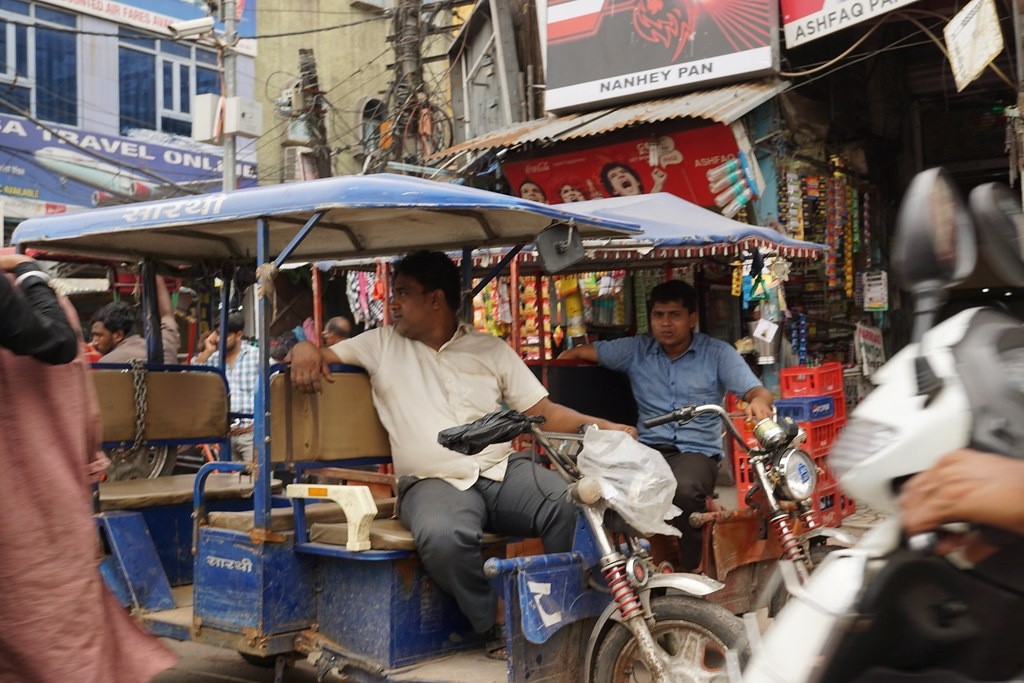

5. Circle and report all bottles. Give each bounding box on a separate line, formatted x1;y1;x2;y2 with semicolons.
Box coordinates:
714;179;747;208
709;169;744;193
721;187;753;219
707;158;741;182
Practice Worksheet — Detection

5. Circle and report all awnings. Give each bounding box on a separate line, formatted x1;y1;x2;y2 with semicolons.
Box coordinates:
421;79;791;161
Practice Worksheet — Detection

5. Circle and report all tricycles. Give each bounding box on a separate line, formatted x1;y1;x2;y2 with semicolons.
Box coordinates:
5;171;859;683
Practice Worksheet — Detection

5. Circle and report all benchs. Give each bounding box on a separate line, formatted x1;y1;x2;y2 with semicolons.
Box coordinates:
205;495;401;538
86;362;284;506
273;368;536;554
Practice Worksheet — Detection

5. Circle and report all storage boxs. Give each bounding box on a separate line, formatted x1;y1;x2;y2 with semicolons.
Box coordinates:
725;360;857;535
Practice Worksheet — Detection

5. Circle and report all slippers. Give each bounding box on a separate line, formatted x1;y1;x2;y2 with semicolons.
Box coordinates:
484;630;508;661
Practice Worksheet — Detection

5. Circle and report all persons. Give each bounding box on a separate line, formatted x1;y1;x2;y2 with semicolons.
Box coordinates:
557;278;774;575
91;260;351;476
0;234;183;683
291;246;637;663
895;449;1024;535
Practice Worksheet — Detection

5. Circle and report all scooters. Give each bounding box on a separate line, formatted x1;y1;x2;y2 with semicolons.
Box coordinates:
735;165;1024;683
